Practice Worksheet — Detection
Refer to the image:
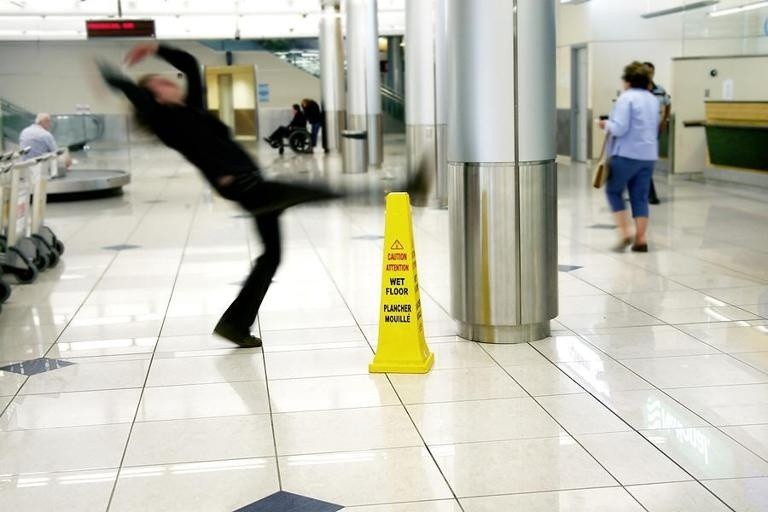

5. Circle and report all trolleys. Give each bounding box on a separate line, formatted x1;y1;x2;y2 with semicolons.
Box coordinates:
0;138;87;312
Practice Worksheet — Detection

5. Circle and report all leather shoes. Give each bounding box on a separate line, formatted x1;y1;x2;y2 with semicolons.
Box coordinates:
214;324;262;347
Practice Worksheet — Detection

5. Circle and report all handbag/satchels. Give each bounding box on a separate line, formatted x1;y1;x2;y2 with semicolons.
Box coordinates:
594;132;610;188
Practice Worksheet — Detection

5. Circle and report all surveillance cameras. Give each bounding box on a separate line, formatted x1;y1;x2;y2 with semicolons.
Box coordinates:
235;29;240;40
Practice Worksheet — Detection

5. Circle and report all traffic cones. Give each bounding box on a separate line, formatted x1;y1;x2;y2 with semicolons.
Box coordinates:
366;190;435;375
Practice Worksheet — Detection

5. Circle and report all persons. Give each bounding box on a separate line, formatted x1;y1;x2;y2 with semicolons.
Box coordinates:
263;104;307;143
643;62;671;205
597;60;661;252
301;99;321;154
94;42;431;349
18;112;71;179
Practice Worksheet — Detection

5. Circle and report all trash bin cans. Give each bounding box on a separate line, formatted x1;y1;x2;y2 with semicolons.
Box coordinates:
341;130;367;175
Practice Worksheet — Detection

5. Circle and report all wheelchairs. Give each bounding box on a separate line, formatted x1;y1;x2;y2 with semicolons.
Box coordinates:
273;119;319;155
